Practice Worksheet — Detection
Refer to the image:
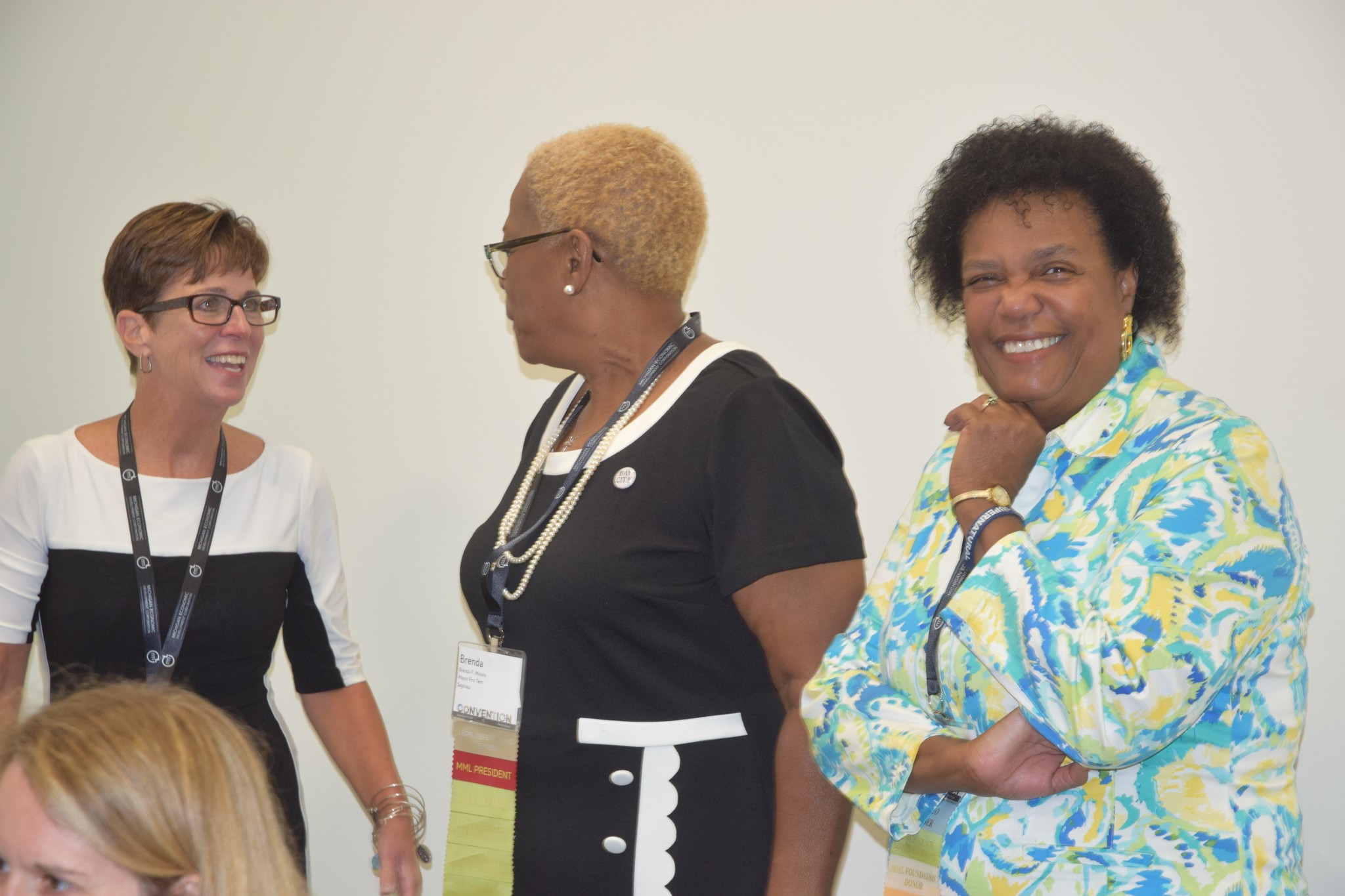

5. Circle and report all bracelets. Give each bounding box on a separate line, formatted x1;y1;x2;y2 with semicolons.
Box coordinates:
368;781;429;852
963;504;1026;573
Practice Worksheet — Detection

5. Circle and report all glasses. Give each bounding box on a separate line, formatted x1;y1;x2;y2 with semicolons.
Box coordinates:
483;230;603;279
135;293;284;327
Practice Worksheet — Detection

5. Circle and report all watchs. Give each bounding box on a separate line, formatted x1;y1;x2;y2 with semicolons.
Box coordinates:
948;483;1012;521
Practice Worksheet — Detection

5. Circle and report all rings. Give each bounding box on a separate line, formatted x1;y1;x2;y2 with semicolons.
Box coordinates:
980;395;998;412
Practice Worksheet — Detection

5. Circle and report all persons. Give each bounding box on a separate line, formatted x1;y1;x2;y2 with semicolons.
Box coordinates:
801;114;1313;896
0;203;431;895
0;679;312;896
456;129;873;896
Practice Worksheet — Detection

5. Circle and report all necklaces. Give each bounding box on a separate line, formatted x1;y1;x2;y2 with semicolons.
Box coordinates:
489;308;702;602
